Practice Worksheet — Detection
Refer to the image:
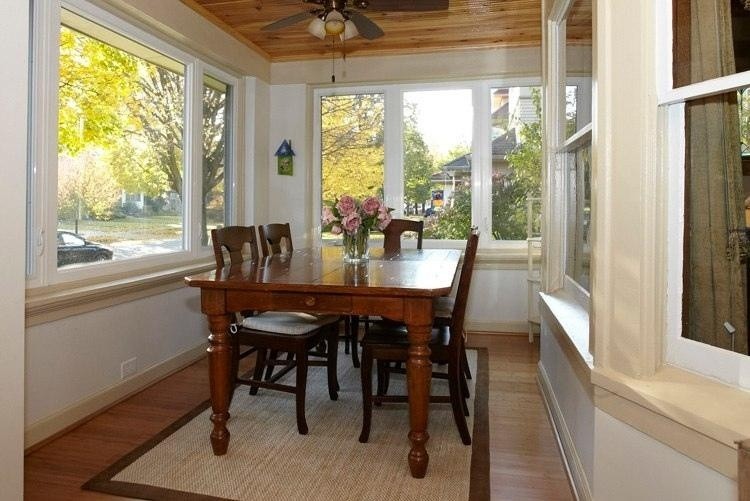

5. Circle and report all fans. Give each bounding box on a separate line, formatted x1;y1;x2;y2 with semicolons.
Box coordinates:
255;0;449;49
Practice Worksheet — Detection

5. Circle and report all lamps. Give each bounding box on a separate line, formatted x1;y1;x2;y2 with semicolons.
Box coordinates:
309;9;355;45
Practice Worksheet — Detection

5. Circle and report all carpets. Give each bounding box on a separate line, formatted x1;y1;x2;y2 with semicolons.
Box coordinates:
79;345;491;499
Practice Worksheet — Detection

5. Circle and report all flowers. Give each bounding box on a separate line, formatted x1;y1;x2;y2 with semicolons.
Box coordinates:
319;196;390;234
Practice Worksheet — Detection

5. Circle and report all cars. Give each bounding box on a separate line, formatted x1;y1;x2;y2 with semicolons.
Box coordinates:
54;227;115;270
403;199;448;220
